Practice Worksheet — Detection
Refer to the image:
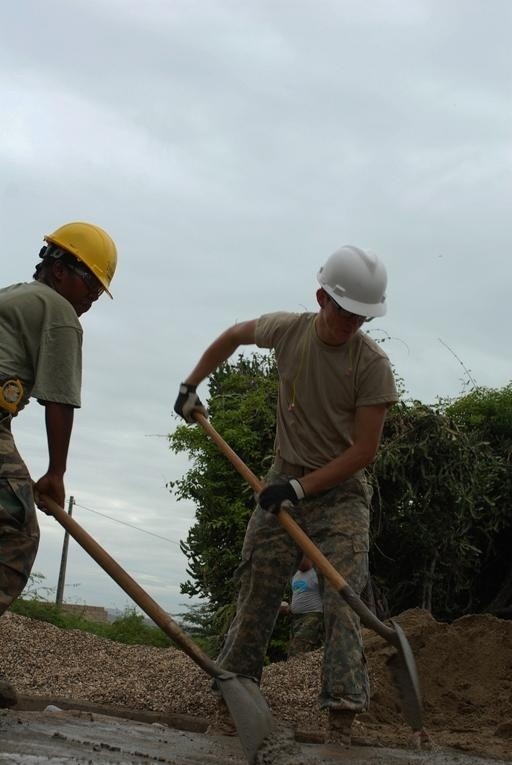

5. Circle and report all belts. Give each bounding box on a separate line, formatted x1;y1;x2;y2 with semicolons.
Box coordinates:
274;456;313;476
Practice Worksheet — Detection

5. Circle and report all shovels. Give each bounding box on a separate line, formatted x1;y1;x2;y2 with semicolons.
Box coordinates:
37;492;274;765
194;410;423;731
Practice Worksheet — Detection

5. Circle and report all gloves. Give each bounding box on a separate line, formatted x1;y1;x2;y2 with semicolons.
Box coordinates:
174;383;208;422
260;479;305;513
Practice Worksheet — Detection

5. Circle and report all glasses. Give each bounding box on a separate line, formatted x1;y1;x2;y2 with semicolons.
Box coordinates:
68;264;103;296
329;295;373;322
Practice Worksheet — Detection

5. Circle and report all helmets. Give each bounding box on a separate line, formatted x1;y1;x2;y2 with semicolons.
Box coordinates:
317;245;386;318
43;222;117;299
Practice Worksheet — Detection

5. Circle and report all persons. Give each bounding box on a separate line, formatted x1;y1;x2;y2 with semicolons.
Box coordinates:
170;240;399;753
1;218;120;712
279;553;326;663
